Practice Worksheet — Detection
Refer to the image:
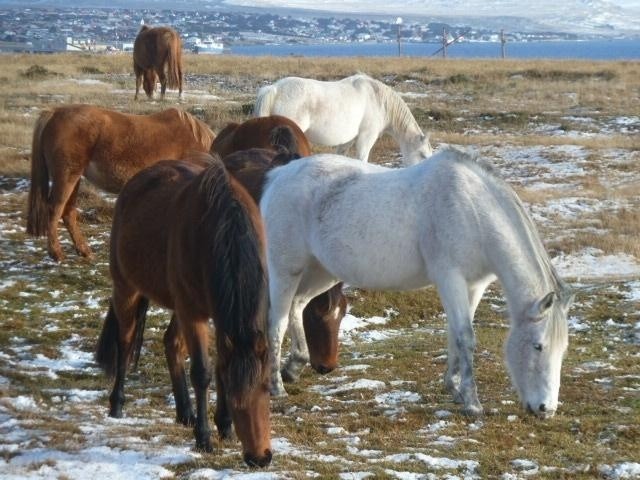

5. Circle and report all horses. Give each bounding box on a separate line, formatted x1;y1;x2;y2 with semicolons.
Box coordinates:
204;115;348;375
259;145;580;419
92;153;273;468
24;105;217;262
133;25;184;101
254;70;433;167
